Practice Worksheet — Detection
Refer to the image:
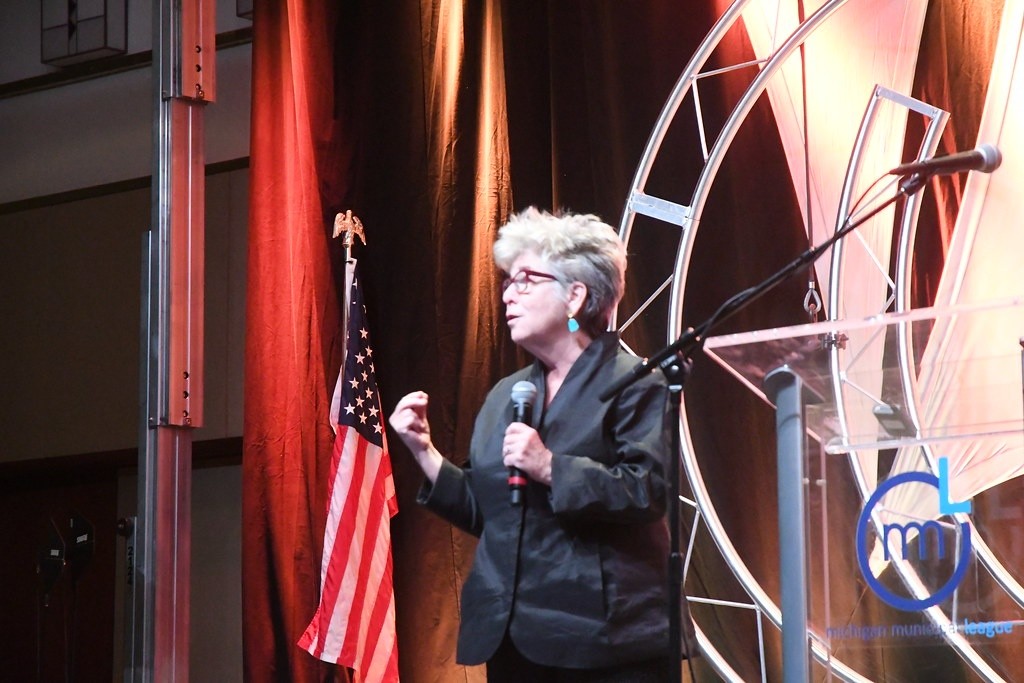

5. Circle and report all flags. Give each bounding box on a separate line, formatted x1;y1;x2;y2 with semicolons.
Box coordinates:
292;254;403;683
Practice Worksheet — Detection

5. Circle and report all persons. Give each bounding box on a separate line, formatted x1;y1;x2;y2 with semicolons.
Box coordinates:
389;203;679;682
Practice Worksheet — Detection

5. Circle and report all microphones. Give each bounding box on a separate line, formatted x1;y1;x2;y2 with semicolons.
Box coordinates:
508;380;537;505
890;144;1003;174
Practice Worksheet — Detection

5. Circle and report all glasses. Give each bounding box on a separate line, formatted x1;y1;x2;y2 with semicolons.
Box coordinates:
503;269;557;295
